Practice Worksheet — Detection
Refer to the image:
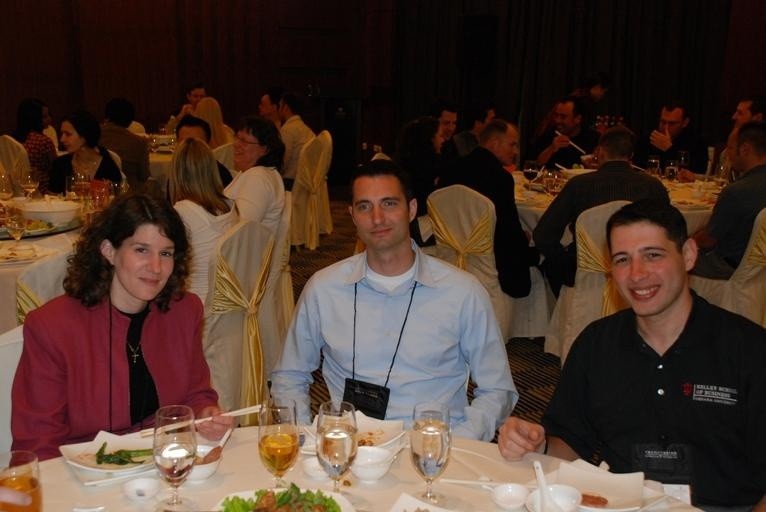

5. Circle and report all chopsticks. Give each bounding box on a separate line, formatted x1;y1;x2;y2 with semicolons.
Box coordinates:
139;404;262;439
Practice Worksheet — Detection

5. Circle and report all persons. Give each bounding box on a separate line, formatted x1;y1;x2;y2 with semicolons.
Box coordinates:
11;97;72;196
162;139;236;306
687;120;766;280
442;103;495;160
496;197;766;511
397;117;445;215
269;161;520;441
532;73;609;157
176;114;232;189
278;93;316;191
258;91;282;128
224;115;285;238
98;97;151;194
170;83;205;122
439;106;457;137
9;191;237;469
533;125;670;300
43;114;68;157
650;99;726;175
195;96;235;150
536;96;601;169
719;96;766;180
46;111;122;191
437;119;539;299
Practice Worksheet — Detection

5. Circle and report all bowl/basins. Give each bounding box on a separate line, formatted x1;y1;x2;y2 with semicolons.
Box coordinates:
123;478;159;502
526;484;582;511
19;201;80;225
490;483;528;510
350;446;394;485
188;444;223;481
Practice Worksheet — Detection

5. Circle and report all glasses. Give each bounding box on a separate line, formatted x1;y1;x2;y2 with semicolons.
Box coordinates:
232;133;259;145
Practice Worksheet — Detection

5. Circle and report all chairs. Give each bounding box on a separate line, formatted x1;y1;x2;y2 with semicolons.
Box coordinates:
16;253;73;324
354;152;392;254
290;138;323;254
0;134;40;196
258;191;295;382
317;131;334;235
687;207;766;328
201;222;277;426
0;325;23;467
212;142;234;170
543;200;634;370
426;184;558;345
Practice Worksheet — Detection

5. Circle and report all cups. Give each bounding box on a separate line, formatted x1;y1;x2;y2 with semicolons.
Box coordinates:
154;404;198;512
409;401;452;507
257;397;300;489
523;163;538;192
6;202;26;248
314;400;358;499
648;156;659;174
664;162;676;184
0;450;42;512
677;151;689;169
21;172;38;200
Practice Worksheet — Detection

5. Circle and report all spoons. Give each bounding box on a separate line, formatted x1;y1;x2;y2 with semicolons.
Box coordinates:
533;461;559;512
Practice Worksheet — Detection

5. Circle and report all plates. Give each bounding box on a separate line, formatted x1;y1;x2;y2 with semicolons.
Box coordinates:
1;244;58;266
67;431;161;473
304;410;405;447
542;458;644;512
384;492;450;512
58;427;157;486
211;489;355;511
524;458;668;510
300;410;404;458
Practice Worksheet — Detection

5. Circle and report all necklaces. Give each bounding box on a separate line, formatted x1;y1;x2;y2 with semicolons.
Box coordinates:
126;340;143;364
76;154;98;173
